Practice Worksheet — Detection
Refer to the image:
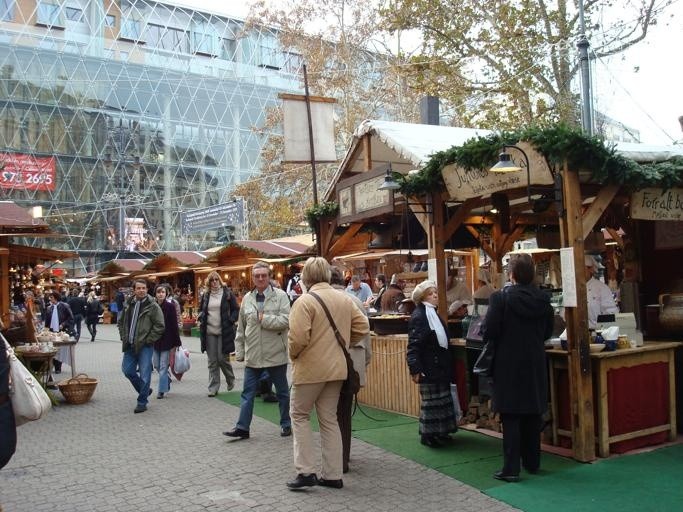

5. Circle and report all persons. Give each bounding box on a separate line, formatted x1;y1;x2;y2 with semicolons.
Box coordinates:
105;297;118;324
223;255;372;489
114;270;240;413
23;287;105;373
347;274;406;312
405;253;617;482
0;333;16;470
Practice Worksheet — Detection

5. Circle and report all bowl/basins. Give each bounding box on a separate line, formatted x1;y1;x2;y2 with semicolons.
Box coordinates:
589;343;605;353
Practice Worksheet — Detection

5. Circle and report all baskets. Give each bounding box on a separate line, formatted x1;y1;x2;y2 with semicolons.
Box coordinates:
57;373;97;404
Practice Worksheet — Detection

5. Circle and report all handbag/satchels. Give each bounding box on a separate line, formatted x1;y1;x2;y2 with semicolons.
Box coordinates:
60;323;76;337
341;356;360;396
0;332;51;427
472;288;504;377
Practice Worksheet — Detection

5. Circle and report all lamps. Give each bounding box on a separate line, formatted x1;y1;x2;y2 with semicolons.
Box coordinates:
486;140;567;224
374;164;437;232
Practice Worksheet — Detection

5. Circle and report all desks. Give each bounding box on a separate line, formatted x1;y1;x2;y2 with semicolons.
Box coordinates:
12;345;61;397
47;335;82;383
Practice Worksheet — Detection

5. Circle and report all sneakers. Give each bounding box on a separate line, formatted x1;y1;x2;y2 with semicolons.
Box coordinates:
208;390;217;396
227;379;235;391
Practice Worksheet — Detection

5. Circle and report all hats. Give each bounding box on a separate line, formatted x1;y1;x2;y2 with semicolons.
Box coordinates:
450;300;468;315
585;256;593;267
411;279;437;306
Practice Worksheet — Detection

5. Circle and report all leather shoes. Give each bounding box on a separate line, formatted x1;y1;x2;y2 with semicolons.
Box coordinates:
222;426;249;438
156;392;163;399
493;470;518;481
437;433;452;442
263;391;278;402
280;425;290;436
317;477;342;489
421;433;453;448
286;473;316;487
134;405;148;413
167;376;171;390
